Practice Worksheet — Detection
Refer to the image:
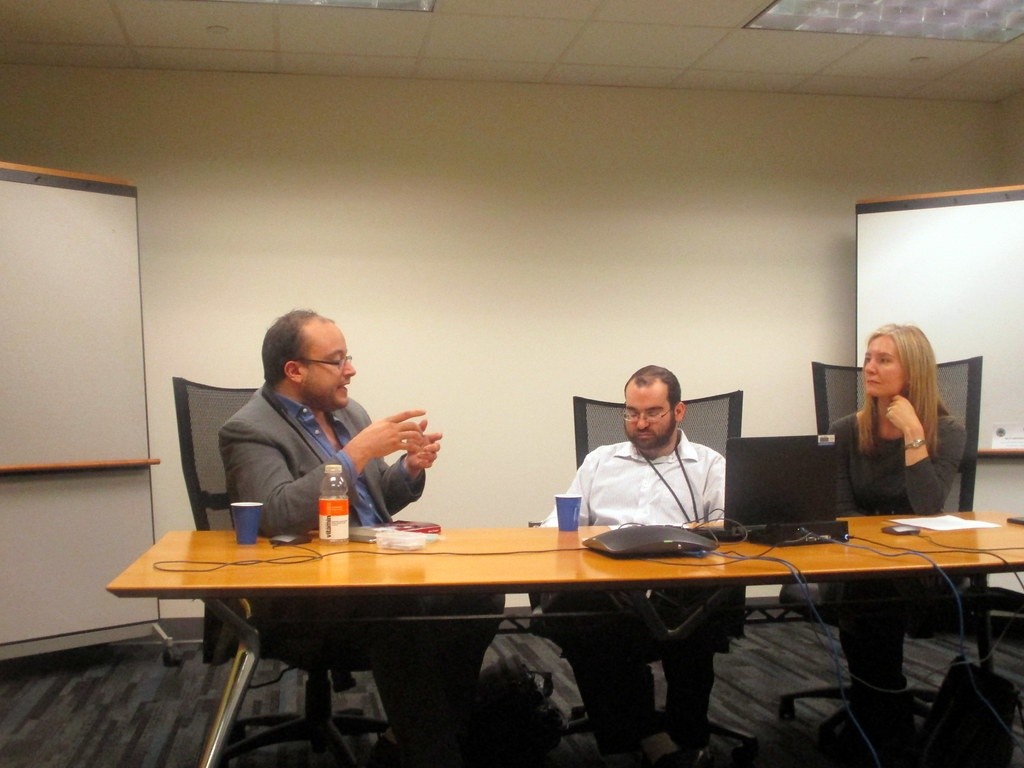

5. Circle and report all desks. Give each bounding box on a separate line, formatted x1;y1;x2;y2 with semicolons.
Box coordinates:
104;512;1024;768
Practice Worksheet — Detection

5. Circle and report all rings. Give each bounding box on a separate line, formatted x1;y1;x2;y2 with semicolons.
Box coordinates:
887;407;891;411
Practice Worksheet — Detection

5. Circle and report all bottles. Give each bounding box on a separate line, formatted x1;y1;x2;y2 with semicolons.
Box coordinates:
318;464;350;544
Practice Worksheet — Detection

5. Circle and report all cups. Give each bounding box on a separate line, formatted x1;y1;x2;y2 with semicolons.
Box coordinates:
230;501;264;545
555;494;582;532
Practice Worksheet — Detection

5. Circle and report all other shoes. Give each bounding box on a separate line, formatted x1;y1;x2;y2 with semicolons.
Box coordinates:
656;748;712;768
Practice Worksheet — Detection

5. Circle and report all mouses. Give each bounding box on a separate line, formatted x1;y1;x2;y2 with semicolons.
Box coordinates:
880;524;921;535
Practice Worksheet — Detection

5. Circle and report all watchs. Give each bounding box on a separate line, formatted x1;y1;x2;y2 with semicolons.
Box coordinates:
904;439;926;450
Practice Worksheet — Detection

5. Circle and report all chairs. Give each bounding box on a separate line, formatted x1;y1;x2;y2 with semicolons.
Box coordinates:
173;376;395;768
559;391;758;768
778;357;982;751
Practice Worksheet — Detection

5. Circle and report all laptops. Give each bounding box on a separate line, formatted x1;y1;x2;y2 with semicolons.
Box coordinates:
690;434;851;539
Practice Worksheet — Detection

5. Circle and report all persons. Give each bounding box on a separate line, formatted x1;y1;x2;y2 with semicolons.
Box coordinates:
538;364;726;768
827;323;965;746
217;310;505;768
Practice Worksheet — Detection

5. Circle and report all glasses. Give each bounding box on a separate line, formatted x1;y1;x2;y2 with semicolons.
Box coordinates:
293;355;353;372
620;405;672;423
